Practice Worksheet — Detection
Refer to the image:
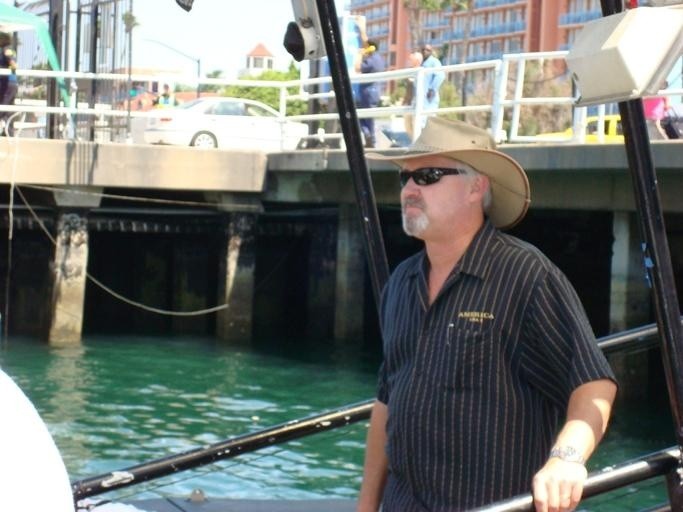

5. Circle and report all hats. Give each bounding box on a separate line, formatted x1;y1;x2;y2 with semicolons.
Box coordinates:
364;115;531;230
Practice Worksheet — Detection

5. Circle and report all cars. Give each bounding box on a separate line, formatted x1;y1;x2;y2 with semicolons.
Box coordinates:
144;96;308;149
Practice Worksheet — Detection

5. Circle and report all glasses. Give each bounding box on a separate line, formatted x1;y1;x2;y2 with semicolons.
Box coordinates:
399;167;468;185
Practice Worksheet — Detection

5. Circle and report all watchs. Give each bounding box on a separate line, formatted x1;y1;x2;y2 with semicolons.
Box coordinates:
548;445;587;467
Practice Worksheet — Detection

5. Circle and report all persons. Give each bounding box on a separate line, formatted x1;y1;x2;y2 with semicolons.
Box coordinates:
401;50;423;140
354;117;615;512
154;80;179;107
0;31;18;137
355;16;385;148
413;44;446;130
